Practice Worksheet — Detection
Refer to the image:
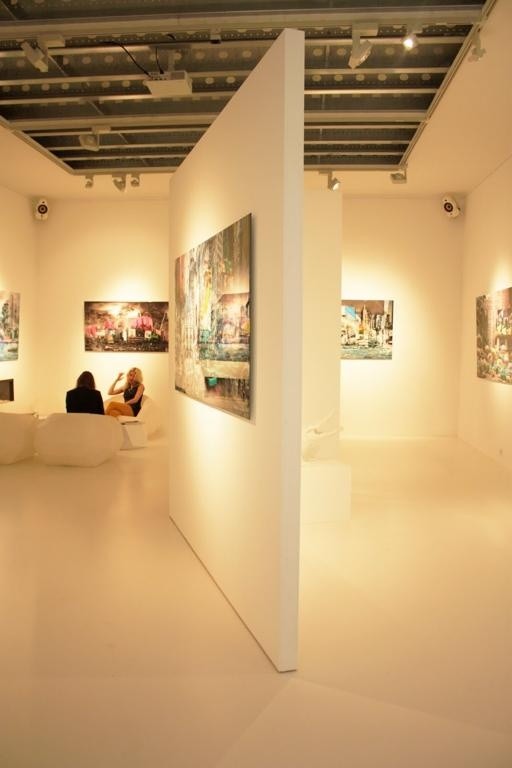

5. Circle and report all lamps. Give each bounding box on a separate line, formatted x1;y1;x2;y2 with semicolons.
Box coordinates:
390;166;407;185
78;124;111;153
467;34;487;63
319;167;341;192
20;32;66;73
84;171;142;194
347;22;379;70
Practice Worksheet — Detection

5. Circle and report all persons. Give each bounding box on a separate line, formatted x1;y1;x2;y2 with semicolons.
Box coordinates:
66;371;106;416
106;367;145;418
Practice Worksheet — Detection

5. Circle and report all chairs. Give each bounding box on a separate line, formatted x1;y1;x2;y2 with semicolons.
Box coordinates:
0;391;164;468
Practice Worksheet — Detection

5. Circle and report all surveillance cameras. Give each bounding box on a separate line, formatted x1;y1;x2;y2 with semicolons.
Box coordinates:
34;198;49;221
441;195;460;218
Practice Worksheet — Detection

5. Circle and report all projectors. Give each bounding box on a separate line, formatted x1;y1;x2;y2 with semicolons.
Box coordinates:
144;70;193;99
391;173;405;184
